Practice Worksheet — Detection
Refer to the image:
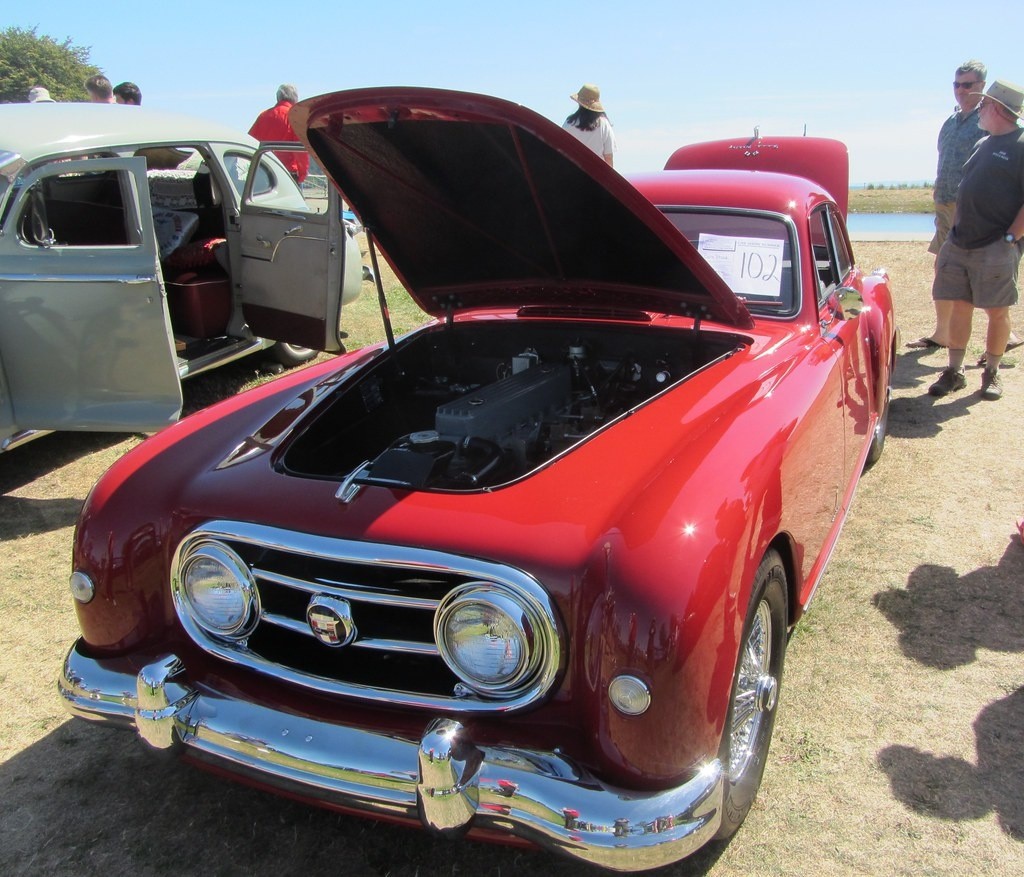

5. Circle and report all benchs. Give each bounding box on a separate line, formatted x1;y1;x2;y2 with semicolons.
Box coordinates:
148;205;232;342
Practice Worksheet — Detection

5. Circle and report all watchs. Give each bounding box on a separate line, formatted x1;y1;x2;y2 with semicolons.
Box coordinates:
1003;231;1017;244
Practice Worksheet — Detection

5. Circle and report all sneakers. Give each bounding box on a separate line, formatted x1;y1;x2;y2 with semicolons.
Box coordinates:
981;367;1003;400
928;365;967;396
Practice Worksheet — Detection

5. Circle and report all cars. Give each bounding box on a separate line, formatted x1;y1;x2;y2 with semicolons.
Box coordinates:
2;98;361;453
63;81;897;869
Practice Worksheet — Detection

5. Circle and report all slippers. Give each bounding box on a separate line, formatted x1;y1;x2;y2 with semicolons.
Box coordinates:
977;340;1024;363
906;336;949;348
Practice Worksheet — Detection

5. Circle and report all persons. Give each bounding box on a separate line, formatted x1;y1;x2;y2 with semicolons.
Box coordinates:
562;83;616;171
85;74;114;104
26;86;57;103
246;83;310;190
904;59;1024;368
926;77;1023;401
111;81;141;106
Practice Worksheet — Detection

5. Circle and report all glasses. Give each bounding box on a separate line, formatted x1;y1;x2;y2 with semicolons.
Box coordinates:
953;81;983;89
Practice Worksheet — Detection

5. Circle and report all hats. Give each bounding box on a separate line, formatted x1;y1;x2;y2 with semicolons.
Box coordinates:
570;83;606;112
29;87;56;102
969;78;1024;120
